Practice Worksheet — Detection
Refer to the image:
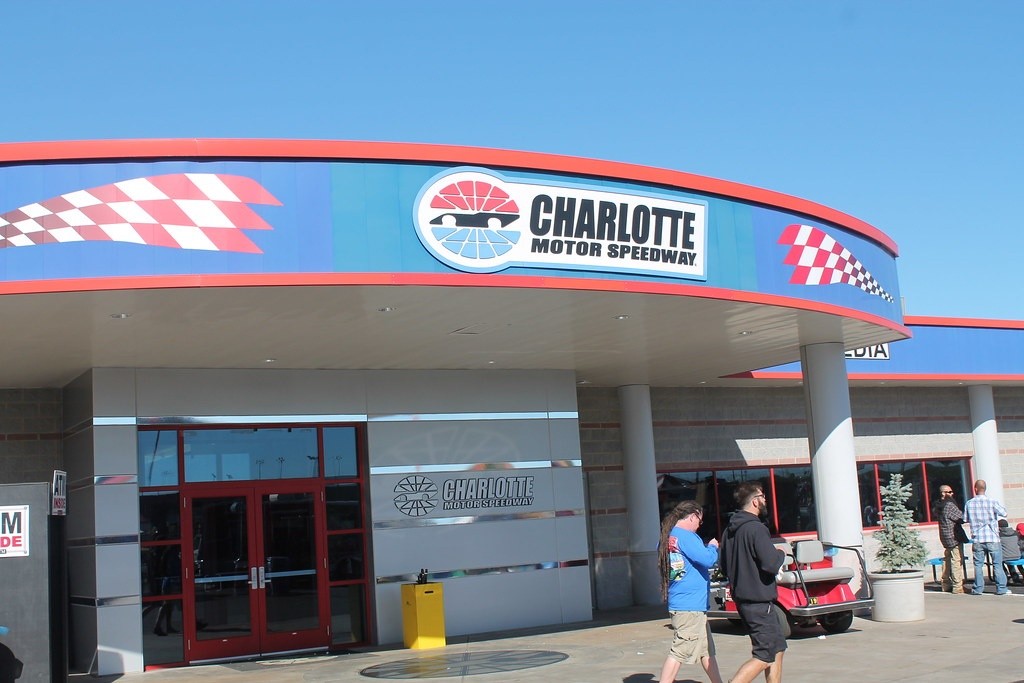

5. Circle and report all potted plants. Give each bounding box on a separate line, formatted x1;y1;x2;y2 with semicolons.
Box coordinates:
867;473;929;622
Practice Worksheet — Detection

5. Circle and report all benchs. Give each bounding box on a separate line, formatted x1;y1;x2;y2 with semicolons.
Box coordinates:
1006;558;1024;566
775;541;853;588
925;555;968;584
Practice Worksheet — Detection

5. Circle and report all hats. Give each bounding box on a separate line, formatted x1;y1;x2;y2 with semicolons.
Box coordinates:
1016;523;1024;536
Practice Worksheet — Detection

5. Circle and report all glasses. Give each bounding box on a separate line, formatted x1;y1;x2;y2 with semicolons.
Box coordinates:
945;491;954;494
690;512;704;526
753;493;766;499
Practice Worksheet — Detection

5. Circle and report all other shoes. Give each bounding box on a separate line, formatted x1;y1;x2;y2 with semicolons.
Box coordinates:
1002;589;1013;595
971;589;982;595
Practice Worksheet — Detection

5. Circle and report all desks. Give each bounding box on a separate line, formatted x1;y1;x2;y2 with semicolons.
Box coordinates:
962;539;1024;582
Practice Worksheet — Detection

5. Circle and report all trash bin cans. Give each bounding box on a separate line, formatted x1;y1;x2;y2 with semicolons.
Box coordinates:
401;582;446;650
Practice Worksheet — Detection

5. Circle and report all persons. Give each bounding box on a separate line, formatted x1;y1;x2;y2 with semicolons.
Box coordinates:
659;501;722;683
936;485;971;594
718;481;787;683
998;519;1022;585
1016;523;1024;581
963;479;1012;595
151;549;208;637
